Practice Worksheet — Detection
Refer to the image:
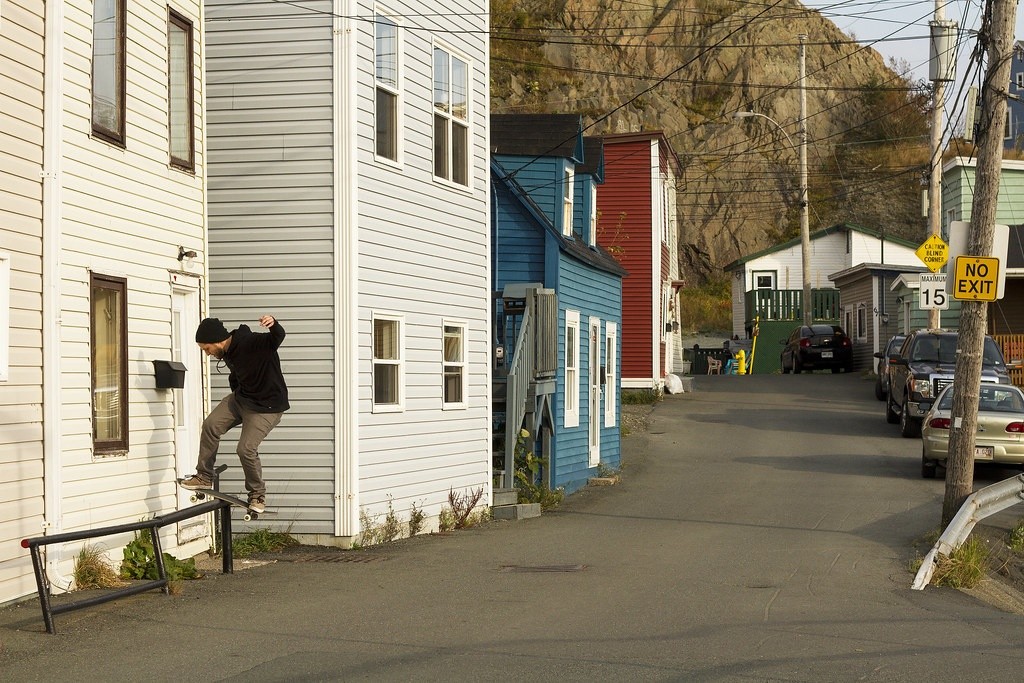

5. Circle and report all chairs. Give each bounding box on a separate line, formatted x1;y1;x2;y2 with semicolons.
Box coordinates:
707;355;722;376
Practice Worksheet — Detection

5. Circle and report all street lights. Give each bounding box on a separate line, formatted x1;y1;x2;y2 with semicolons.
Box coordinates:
735;109;800;161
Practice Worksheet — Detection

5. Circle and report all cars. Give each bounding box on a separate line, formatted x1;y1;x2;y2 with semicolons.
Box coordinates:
780;322;853;374
872;335;908;400
887;329;1016;438
921;380;1024;481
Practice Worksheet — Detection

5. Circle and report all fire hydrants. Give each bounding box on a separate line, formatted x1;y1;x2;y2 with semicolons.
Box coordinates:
735;349;747;375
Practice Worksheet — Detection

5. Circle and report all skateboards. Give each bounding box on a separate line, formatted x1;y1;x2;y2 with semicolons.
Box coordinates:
176;477;279;522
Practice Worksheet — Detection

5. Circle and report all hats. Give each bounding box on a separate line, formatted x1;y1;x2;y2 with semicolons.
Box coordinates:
196;318;230;345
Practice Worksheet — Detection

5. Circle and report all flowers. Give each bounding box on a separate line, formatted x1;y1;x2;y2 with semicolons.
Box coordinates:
513;429;540;505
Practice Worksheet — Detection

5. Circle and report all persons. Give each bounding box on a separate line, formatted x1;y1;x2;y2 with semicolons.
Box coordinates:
180;315;292;512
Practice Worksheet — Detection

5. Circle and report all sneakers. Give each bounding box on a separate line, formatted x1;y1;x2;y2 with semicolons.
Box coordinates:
181;477;212;491
248;498;265;514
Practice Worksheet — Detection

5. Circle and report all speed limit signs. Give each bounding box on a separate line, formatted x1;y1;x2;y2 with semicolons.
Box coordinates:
920;272;950;311
